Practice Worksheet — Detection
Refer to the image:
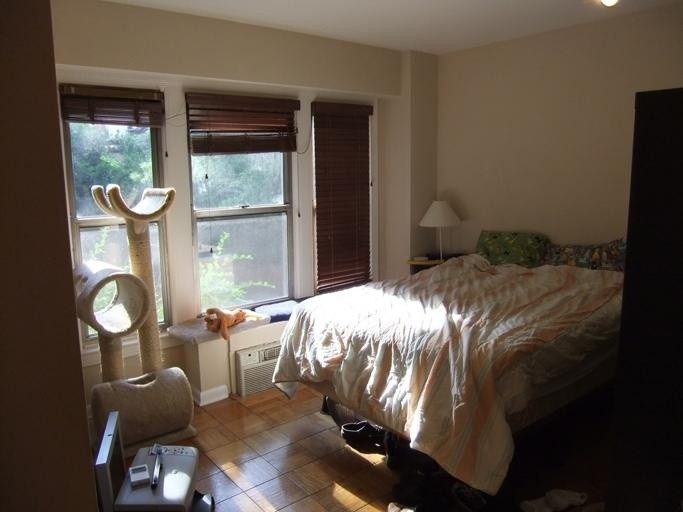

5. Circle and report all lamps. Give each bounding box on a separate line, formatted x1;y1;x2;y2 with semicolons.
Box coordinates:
418;200;461;262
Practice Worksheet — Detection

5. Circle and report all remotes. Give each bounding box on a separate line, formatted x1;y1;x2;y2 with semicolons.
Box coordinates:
128;463;150;488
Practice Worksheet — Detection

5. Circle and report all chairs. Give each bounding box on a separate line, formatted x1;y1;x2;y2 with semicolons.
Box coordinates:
95;407;215;512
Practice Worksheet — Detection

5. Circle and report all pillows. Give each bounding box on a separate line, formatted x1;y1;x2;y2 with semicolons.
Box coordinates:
473;228;625;272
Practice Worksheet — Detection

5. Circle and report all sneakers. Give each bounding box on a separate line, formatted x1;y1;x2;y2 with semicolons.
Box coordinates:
341;420;384;439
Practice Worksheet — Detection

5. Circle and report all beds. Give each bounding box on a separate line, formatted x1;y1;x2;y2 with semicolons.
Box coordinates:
279;256;624;500
